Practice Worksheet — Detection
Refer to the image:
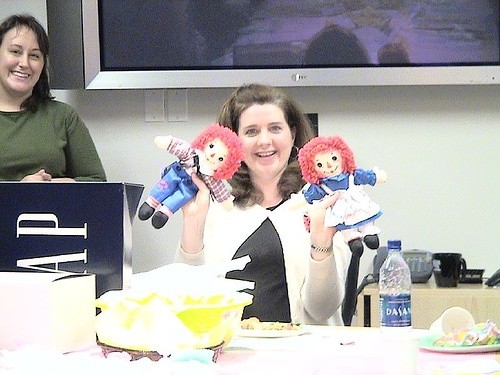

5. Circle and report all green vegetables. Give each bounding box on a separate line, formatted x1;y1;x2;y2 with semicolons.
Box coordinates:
451;336;500;346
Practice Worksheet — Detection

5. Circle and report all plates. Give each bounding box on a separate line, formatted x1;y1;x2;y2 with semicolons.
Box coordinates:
237;322;308;336
420;344;500;353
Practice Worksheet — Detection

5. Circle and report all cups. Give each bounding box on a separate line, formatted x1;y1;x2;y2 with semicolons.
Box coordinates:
429;307;475;337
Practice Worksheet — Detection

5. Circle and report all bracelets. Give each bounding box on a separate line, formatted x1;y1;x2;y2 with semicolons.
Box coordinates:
311;244;332;252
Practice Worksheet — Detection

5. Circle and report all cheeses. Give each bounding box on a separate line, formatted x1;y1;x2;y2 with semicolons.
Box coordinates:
429;306;476;339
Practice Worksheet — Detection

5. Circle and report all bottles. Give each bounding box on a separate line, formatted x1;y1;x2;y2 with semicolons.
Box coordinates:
379;239;412;360
432;253;467;288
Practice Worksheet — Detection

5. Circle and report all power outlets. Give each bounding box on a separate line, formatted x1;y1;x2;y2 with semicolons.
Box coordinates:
168;90;188;122
144;90;164;121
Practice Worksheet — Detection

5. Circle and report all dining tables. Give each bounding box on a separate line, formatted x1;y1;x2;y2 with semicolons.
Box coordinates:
0;323;500;375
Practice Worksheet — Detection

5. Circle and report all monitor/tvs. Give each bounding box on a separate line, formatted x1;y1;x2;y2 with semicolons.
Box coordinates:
44;0;500;91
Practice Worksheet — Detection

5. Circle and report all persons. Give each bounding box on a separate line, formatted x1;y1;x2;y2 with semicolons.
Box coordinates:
0;14;107;183
138;123;245;230
176;84;352;326
289;135;385;256
